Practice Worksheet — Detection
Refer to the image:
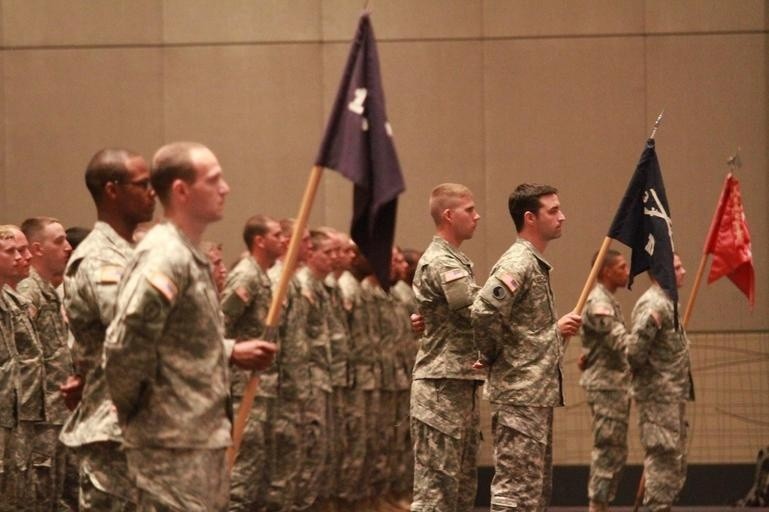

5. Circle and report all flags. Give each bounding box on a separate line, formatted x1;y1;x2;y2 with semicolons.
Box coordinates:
607;138;678;332
313;13;406;293
704;173;755;315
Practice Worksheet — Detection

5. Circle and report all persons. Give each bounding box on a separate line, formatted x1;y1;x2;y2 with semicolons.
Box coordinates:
625;251;695;512
204;215;422;511
578;250;631;512
57;144;156;511
409;182;582;512
0;216;92;511
103;141;232;511
471;182;567;512
